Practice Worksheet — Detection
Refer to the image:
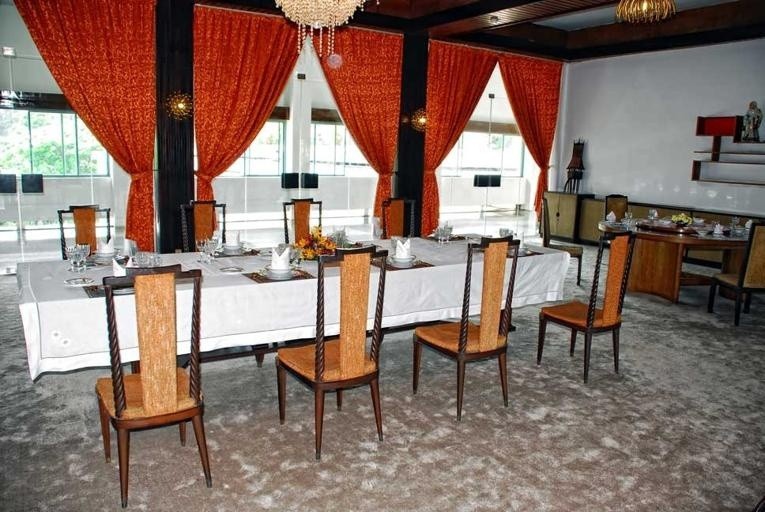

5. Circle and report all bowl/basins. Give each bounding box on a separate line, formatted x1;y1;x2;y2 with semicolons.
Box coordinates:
391;255;415;262
222;242;243;251
698;230;708;236
265;264;294;273
96;249;115;256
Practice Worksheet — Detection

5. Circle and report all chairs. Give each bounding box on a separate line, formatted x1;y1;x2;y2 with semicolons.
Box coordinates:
382;198;414;239
283;199;322;243
709;223;765;326
602;194;629;251
414;235;520;420
538;231;635;385
183;200;225;251
58;205;111;260
96;264;213;507
275;244;388;460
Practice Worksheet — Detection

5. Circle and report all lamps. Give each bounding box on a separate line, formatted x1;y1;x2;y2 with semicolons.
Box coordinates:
275;0;379;57
616;0;674;22
165;90;192;119
411;109;427;131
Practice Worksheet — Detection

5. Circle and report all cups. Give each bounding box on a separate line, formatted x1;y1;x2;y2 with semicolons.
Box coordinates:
624;212;632;224
649;210;657;219
499;228;508;238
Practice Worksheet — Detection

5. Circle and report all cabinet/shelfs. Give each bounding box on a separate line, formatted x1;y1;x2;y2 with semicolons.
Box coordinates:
540;191;595;244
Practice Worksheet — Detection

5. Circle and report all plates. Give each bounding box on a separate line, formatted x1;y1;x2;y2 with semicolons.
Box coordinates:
90;254;124;262
64;277;94;286
215;248;252;255
261;270;298;279
